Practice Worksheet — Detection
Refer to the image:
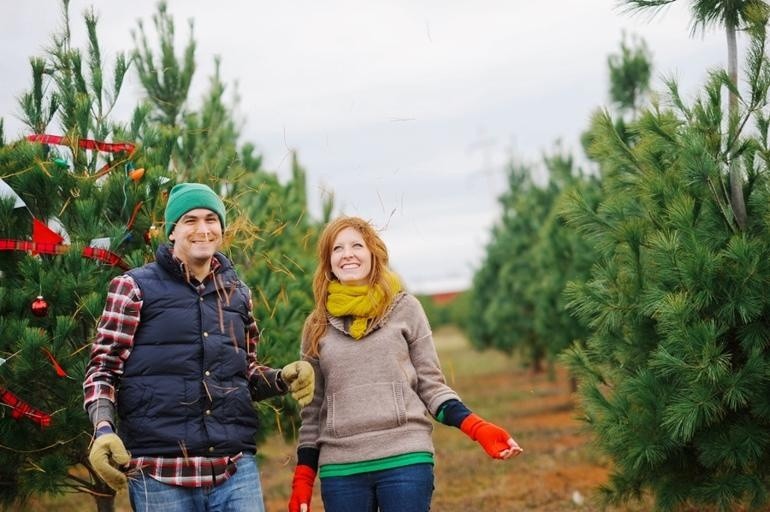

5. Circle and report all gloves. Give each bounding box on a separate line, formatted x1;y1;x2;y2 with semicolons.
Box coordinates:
281;360;315;406
89;426;129;490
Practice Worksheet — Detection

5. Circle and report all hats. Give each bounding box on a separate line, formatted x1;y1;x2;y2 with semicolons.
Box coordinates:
165;183;226;239
